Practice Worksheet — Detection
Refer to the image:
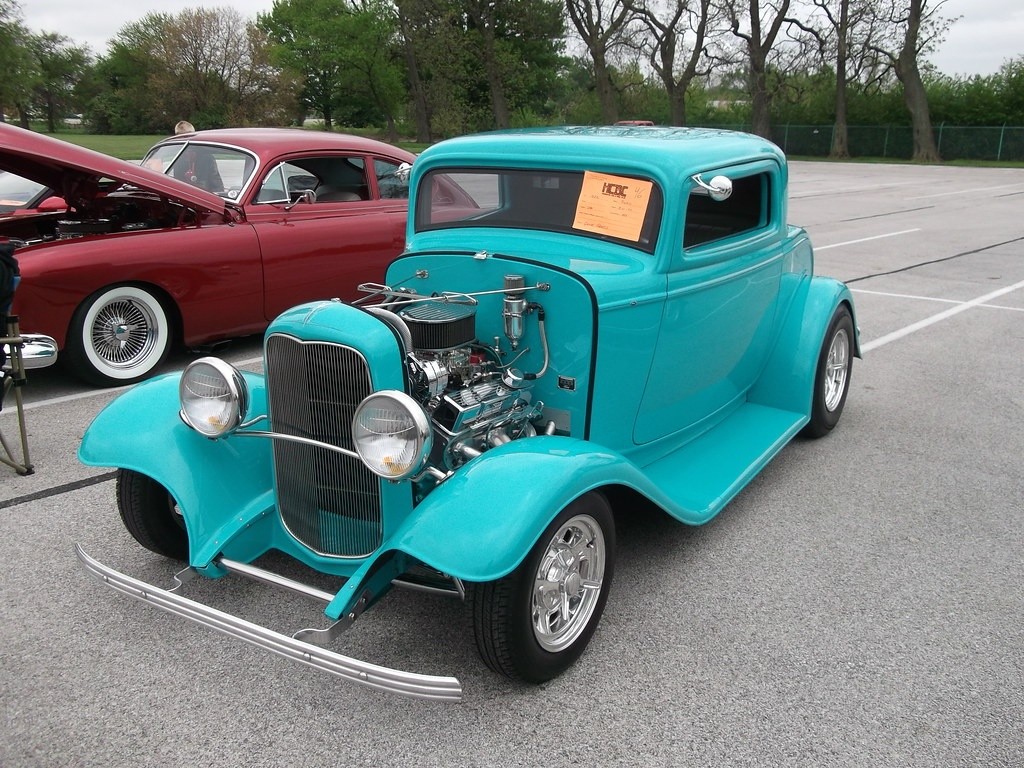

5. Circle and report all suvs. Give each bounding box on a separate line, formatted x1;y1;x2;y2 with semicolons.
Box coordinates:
72;124;865;705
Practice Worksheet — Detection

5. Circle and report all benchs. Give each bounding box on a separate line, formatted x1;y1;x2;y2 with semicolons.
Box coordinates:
683;212;755;245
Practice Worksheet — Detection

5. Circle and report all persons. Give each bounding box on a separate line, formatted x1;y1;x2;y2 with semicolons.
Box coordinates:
174;120;225;192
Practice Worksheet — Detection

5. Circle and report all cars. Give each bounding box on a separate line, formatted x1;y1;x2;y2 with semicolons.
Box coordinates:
0;121;497;383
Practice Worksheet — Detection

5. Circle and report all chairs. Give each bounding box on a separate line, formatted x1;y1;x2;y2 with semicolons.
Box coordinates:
261;185;286;201
318;191;360;201
